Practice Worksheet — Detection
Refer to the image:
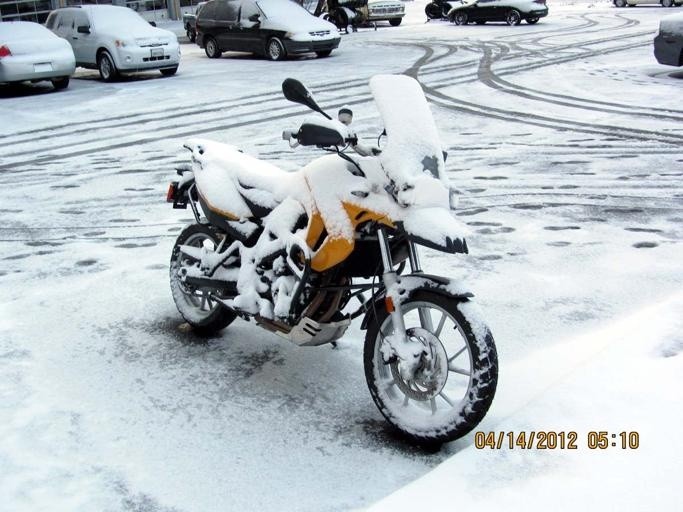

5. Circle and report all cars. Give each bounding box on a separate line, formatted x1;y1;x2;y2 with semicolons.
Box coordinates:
653;10;683;66
446;0;548;26
358;1;406;27
0;19;75;92
612;0;683;7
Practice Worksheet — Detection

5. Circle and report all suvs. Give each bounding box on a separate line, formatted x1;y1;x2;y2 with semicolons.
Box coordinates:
41;6;180;83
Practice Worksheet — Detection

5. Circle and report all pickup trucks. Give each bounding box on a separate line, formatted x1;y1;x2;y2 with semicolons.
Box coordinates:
183;2;206;41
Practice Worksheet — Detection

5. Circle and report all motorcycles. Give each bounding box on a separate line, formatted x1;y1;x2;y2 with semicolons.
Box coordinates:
166;72;499;448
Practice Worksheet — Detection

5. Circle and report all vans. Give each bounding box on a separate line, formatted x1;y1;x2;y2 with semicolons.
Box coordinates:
195;0;341;61
